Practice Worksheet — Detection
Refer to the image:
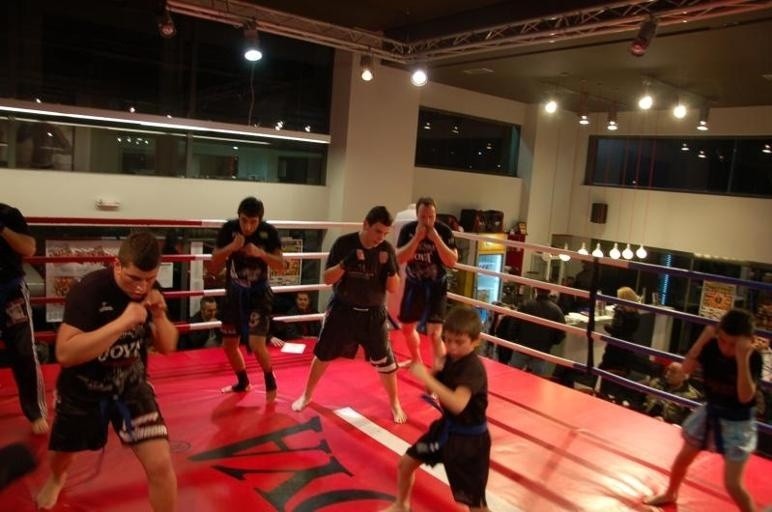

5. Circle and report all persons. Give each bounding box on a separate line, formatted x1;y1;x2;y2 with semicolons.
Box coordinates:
0;197;49;435
268;334;285;348
35;232;180;512
642;306;764;512
289;292;322;337
208;196;283;406
379;304;491;512
396;197;458;373
179;296;223;350
495;281;772;458
291;205;408;424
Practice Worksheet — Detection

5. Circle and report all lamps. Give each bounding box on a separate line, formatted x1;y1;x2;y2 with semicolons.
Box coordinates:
360;58;376;83
670;92;688;120
158;11;177;40
540;71;662;263
628;15;657;57
695;101;710;132
408;59;432;88
241;26;263;62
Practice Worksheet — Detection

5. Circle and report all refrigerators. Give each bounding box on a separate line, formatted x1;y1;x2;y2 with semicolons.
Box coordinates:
451;232;509;323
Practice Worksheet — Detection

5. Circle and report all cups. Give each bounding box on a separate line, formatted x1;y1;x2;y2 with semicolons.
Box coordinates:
642;284;659;305
596;300;606;311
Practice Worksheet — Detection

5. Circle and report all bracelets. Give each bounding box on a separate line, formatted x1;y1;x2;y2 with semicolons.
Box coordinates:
0;220;4;233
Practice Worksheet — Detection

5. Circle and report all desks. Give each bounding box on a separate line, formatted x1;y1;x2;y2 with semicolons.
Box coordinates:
564;303;676;368
